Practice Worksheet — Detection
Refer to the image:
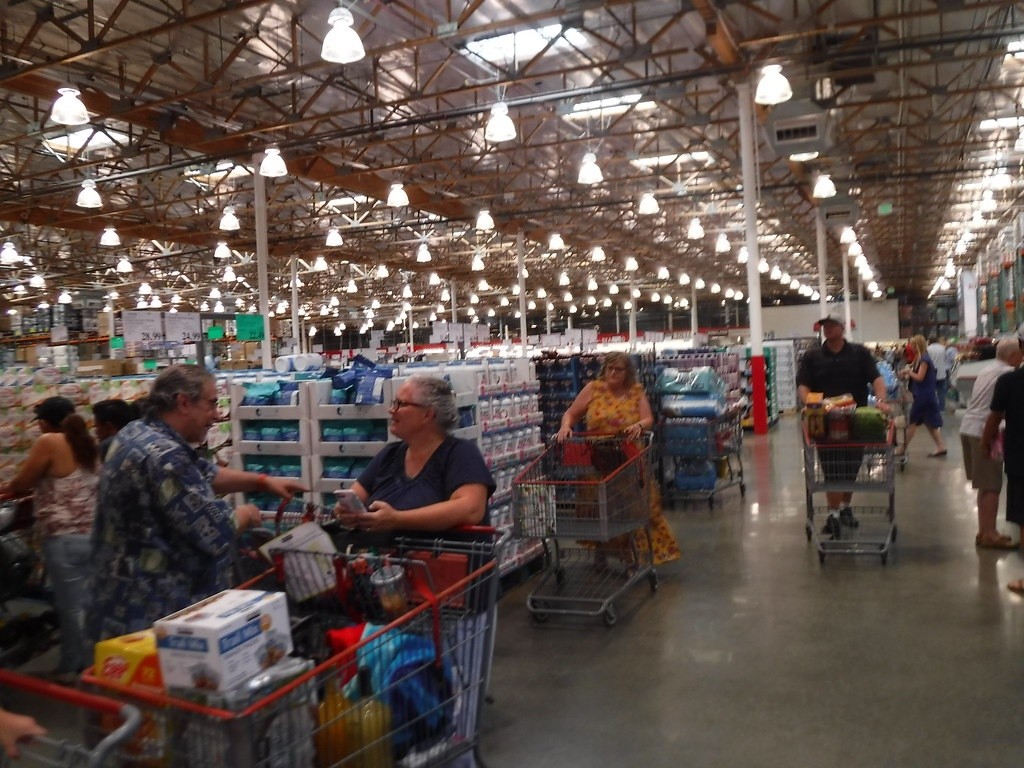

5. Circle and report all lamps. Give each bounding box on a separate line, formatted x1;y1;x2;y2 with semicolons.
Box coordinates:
753;0;882;299
259;0;747;330
0;14;255;313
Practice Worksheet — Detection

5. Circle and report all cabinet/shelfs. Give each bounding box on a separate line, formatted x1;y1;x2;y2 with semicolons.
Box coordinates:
231;346;779;616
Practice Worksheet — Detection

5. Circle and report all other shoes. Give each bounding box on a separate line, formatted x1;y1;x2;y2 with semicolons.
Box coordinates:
42;666;77;683
823;514;839;536
1007;577;1024;594
840;508;858;527
928;450;947;458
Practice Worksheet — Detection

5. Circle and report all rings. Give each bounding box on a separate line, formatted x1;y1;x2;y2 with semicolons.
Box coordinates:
366;528;370;533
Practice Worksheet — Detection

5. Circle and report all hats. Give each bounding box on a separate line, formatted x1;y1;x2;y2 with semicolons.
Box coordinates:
32;397;74;422
819;314;845;328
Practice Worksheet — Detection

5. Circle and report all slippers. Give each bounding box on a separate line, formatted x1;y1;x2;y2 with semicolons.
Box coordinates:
975;532;1018;549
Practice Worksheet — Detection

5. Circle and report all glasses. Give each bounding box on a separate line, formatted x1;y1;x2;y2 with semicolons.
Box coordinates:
390;397;429;411
605;365;625;372
190;395;217;409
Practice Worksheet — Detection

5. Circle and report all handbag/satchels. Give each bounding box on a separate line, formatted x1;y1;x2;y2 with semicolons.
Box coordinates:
589;439;628;475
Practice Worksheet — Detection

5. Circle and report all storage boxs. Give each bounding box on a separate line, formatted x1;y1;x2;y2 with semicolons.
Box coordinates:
807;392;824;440
95;588;319;768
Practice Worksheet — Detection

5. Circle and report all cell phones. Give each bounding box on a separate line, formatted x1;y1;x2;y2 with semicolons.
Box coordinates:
334;489;368;514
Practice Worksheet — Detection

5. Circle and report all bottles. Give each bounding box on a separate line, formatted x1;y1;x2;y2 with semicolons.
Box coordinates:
318;663;395;768
274;353;323;372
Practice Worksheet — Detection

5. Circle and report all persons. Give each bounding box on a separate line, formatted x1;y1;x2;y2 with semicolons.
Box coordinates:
795;312;1024;595
557;351;680;579
0;361;312;755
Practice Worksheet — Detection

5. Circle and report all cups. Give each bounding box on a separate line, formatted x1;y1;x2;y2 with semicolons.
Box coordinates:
370;564;411;628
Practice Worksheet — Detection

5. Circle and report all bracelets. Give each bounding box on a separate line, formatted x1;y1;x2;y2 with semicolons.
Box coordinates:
638;422;644;432
256;474;268;494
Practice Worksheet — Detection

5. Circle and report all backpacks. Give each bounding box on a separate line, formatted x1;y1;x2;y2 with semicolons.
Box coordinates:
327;622;455;763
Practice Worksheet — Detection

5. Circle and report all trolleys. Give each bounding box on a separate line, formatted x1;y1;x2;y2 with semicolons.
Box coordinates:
653;402;746;509
879;383;914;472
510;431;659;627
1;670;142;767
802;412;898;565
233;488;348;585
80;523;512;768
0;491;59;667
